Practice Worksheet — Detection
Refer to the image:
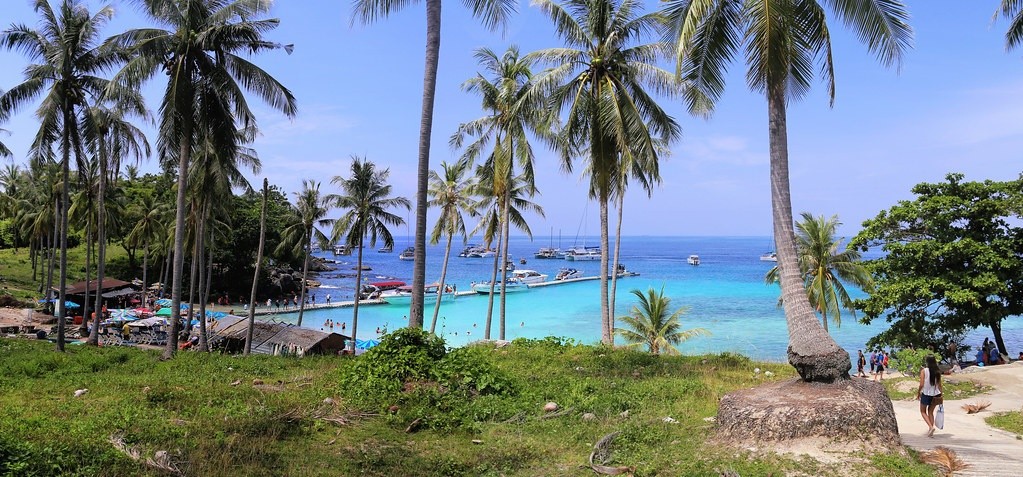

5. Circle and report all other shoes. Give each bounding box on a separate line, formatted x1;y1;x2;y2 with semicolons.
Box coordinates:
927;428;935;437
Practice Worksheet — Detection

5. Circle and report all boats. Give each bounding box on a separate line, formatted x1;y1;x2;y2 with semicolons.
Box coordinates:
379;283;455;305
507;269;548;283
612;263;626;273
519;257;527;264
304;241;365;255
457;244;503;257
377;248;391;253
354;281;406;300
473;277;532;295
687;254;700;265
554;267;584;280
499;254;515;271
760;229;777;261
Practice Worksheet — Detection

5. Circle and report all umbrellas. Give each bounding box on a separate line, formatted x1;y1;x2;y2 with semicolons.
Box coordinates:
39;298;80;310
149;282;164;292
91;298;228;332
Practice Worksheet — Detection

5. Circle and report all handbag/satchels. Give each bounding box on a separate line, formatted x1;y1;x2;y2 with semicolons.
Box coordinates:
861;356;865;365
934;404;944;429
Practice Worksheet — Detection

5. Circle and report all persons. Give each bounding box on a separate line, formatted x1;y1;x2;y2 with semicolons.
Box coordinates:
857;349;891;381
974;337;1005;366
445;280;487;293
102;305;109;319
948;339;957;367
917;353;943;437
319;319;346;331
122;323;130;340
147;292;165;305
218;292;331;311
376;314;525;337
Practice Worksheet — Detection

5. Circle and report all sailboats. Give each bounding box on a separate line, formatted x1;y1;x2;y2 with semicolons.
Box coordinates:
534;226;566;259
565;196;602;261
400;213;414;260
